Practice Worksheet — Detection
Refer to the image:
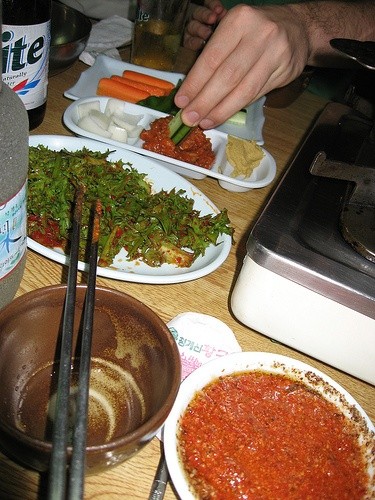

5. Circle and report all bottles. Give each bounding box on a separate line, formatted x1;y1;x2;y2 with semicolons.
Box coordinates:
0;0;55;132
0;76;30;313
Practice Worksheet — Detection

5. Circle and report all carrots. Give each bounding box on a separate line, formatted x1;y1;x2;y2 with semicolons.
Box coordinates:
97;69;173;104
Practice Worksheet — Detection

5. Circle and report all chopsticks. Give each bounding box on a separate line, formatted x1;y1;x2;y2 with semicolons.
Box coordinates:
45;185;101;500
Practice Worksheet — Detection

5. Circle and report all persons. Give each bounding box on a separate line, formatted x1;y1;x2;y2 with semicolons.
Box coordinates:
63;0;375;128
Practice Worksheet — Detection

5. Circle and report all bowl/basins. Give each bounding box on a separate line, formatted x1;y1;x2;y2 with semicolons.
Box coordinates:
49;0;92;78
0;284;181;476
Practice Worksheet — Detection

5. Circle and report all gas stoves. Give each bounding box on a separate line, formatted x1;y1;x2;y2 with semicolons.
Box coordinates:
231;101;375;387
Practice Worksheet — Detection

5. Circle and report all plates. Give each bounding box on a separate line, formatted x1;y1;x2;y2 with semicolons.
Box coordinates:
62;95;278;193
161;350;375;499
26;134;231;284
63;53;267;146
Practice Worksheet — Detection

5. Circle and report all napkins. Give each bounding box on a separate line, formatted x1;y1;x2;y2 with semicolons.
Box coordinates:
79;14;135;66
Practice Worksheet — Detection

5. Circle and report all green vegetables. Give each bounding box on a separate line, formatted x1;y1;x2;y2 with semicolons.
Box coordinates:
134;78;186;114
26;144;236;269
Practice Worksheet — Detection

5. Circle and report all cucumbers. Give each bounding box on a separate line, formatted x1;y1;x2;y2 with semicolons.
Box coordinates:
229;108;247;125
169;108;196;146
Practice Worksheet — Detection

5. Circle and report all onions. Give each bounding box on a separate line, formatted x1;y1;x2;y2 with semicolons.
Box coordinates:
76;99;144;144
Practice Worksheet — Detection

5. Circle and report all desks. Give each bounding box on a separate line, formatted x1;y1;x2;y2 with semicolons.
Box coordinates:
0;34;375;500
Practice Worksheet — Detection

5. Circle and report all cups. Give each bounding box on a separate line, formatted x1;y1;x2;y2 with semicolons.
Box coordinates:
264;65;318;109
129;0;191;72
